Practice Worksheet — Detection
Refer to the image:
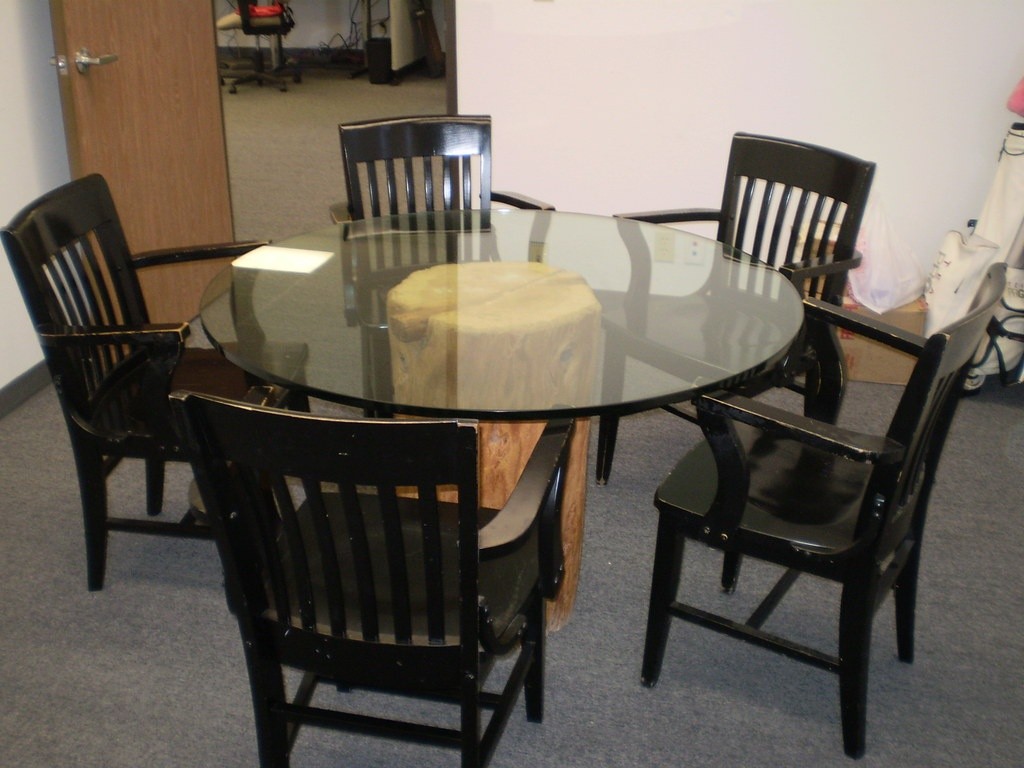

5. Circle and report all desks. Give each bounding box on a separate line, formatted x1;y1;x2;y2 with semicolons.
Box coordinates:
201;210;804;632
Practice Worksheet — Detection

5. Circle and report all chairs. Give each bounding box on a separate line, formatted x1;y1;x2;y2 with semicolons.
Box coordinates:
327;115;555;418
0;172;309;592
640;264;1009;760
168;387;577;768
597;133;876;484
216;0;302;93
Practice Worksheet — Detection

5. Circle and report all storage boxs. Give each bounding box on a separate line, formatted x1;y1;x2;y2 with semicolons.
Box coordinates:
795;217;927;385
